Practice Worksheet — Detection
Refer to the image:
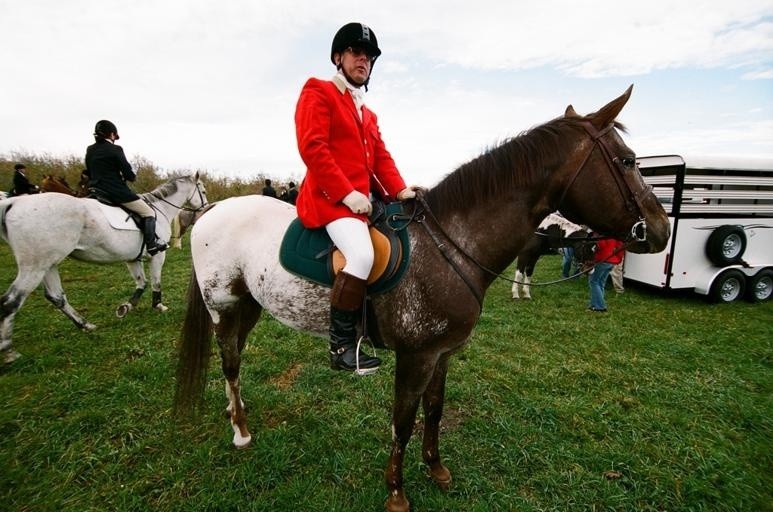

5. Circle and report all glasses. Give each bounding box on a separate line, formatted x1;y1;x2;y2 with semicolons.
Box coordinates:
343;45;375;61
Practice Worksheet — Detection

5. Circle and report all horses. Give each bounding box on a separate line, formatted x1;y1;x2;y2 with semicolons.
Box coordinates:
39;175;76;196
57;175;70;189
171;82;672;511
0;169;210;364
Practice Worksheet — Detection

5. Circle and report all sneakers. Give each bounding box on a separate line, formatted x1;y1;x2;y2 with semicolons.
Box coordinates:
587;304;608;313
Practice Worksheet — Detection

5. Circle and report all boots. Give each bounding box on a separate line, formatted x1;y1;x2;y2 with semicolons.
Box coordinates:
326;304;384;371
140;215;167;257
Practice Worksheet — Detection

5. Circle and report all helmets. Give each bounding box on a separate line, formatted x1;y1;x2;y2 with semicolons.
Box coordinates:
80;169;89;176
328;21;382;67
92;119;120;140
13;163;27;170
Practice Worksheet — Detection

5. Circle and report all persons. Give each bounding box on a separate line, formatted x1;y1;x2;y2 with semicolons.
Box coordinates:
292;21;423;375
608;253;625;301
83;120;166;256
11;163;38;194
562;240;581;282
586;237;626;312
262;178;276;198
287;182;297;205
276;185;287;202
